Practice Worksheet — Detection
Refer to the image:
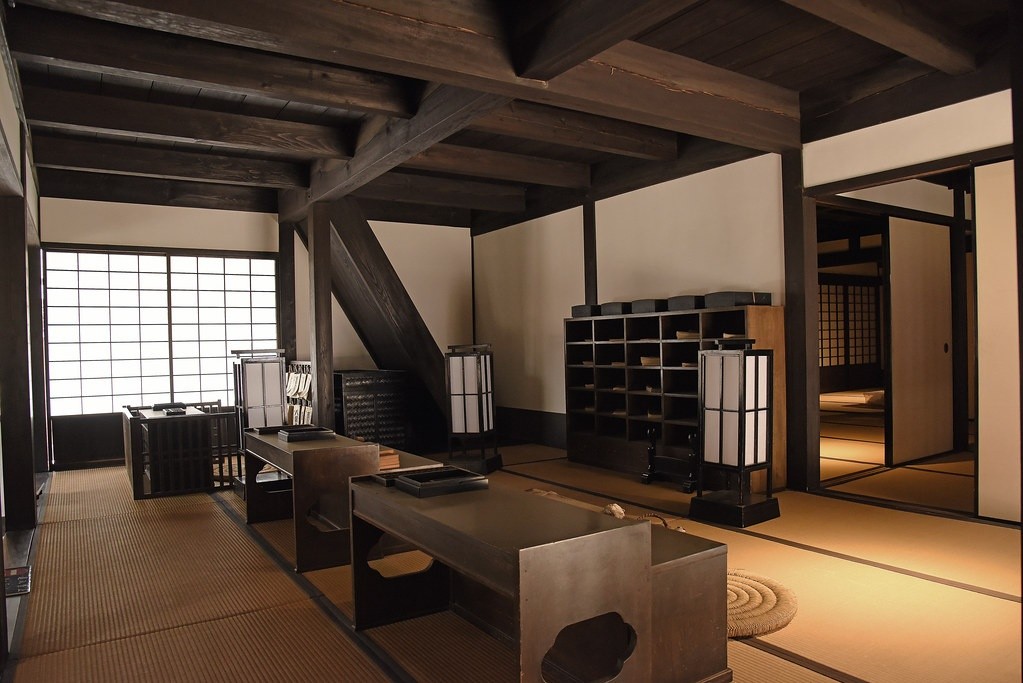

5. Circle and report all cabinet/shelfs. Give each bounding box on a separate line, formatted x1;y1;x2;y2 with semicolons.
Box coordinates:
561;306;787;496
333;369;417;456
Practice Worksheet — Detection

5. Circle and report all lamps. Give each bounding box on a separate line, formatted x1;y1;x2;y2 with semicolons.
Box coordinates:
689;339;782;528
441;343;505;477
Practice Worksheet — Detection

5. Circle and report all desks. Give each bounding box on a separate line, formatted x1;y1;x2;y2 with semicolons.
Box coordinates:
241;424;381;573
138;406;214;494
343;441;735;683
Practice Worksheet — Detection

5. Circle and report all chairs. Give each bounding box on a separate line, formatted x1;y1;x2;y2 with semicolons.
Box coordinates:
192;398;225;464
